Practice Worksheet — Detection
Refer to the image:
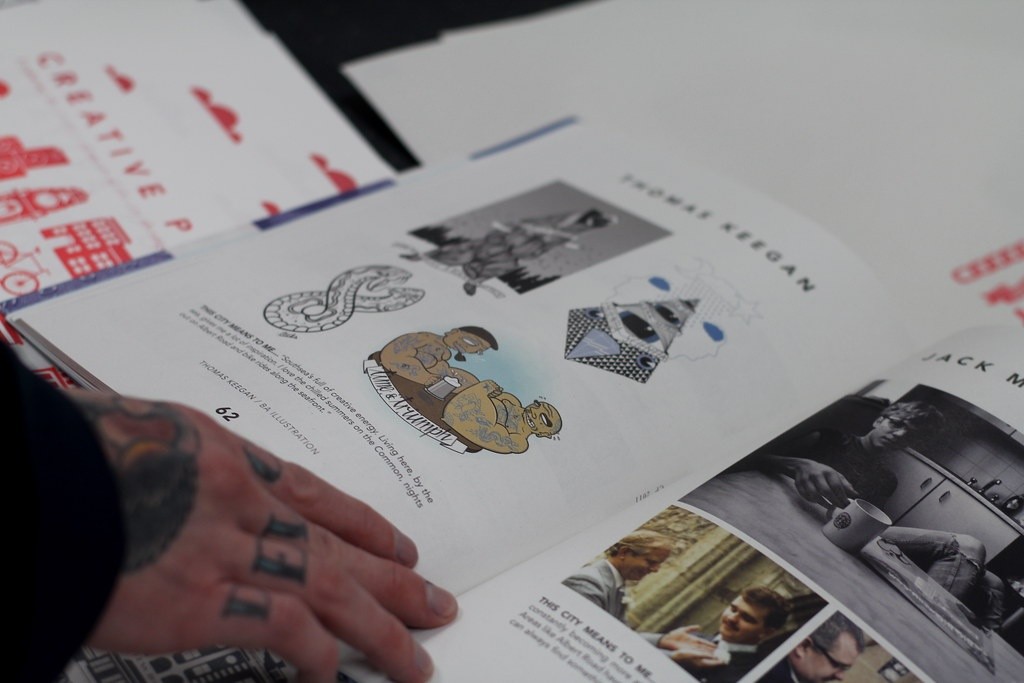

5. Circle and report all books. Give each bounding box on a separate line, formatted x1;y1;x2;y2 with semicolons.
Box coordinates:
0;115;1024;683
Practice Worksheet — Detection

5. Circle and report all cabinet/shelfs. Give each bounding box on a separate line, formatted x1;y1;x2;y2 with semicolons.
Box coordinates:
867;447;1021;570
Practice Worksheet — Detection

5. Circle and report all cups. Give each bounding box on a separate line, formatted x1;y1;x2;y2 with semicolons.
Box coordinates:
821;497;892;556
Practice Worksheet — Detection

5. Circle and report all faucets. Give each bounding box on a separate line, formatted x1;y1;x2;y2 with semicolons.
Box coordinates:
980;478;1002;496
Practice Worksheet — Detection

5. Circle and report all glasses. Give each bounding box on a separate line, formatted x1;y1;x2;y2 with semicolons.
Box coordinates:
811;641;852;674
885;414;920;444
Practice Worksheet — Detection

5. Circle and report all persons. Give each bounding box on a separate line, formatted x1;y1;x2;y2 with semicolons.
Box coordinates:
758;612;865;683
560;529;721;662
677;585;789;683
0;339;458;683
747;401;1003;629
